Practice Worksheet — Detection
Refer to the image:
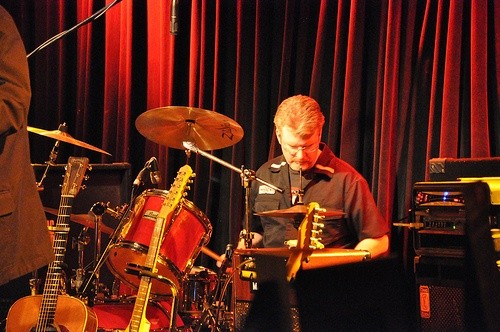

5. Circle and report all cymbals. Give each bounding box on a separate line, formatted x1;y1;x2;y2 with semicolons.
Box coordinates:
40;205;116;235
26;125;112;156
254;204;348;222
135;107;244;151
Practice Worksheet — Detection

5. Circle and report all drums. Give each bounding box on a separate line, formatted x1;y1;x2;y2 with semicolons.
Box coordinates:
87;298;192;332
106;188;212;297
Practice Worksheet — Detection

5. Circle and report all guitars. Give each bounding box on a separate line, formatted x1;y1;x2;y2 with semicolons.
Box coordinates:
284;202;324;283
6;157;100;332
123;165;196;332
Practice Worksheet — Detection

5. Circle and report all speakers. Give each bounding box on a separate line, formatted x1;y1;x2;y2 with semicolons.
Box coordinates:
417;277;500;332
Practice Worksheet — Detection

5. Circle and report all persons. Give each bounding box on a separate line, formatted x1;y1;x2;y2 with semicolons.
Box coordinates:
0;5;55;287
236;94;389;332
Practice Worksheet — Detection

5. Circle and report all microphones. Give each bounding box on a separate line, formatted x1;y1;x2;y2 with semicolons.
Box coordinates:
149;158;162;185
170;0;179;34
